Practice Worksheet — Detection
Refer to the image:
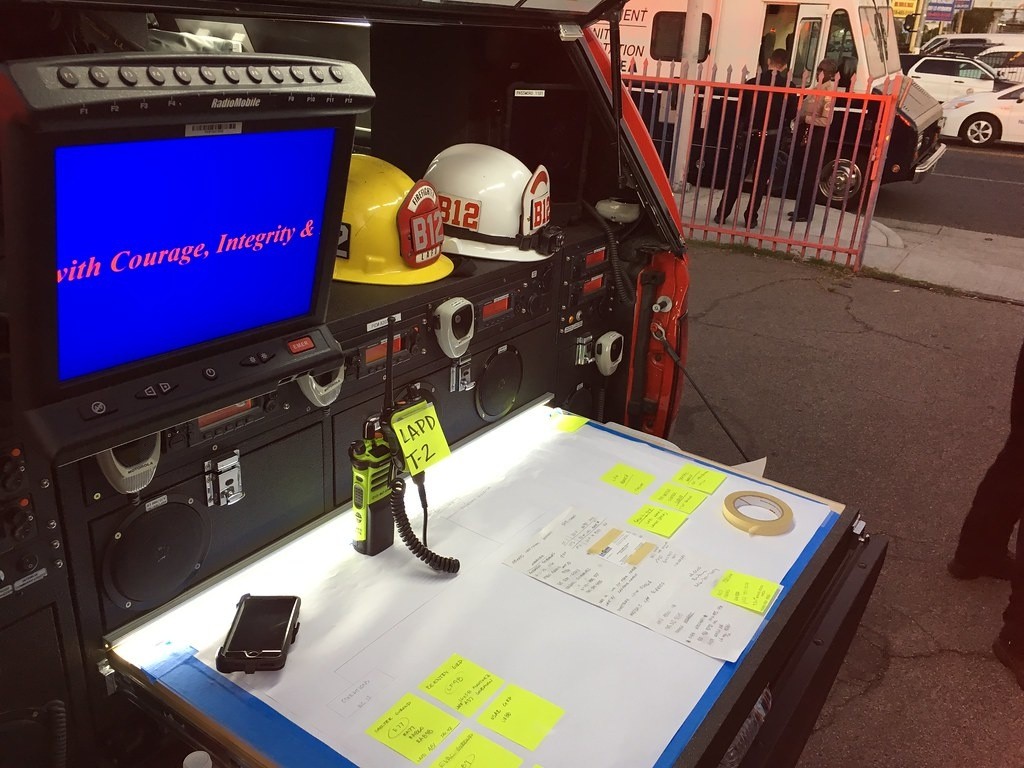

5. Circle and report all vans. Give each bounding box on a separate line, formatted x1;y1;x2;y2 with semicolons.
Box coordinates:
583;2;946;212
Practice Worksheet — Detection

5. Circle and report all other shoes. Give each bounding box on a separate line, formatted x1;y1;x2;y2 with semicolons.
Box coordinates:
992;630;1024;690
745;219;758;228
949;547;1015;579
789;216;809;221
788;211;797;216
714;215;726;224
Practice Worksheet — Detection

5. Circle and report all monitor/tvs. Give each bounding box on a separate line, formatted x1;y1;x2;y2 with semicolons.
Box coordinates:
0;0;377;408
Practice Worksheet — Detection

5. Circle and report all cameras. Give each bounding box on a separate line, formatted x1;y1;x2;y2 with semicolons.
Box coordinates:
216;593;301;674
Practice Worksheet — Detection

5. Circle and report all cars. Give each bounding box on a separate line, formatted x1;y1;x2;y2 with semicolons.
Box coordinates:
897;32;1024;147
1;2;888;767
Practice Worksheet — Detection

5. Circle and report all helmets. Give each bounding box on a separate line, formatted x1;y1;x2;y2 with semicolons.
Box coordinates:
424;143;563;262
333;155;455;285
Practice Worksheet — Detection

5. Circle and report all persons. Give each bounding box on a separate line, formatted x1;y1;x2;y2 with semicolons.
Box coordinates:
947;343;1024;691
714;50;797;228
788;59;839;222
